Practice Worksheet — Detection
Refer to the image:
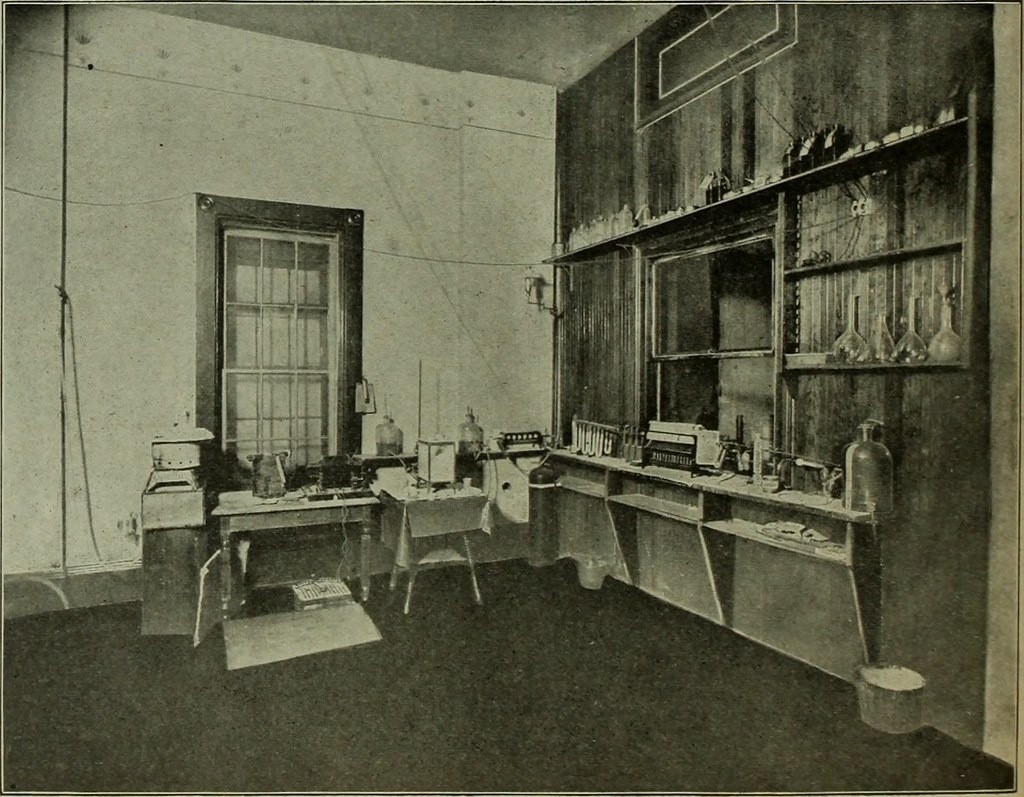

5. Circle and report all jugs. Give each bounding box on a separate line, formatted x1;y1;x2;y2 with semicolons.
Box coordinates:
246;450;290;498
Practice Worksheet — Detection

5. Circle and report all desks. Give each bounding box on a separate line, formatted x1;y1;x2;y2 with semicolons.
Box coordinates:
210;487;383;615
375;467;488;615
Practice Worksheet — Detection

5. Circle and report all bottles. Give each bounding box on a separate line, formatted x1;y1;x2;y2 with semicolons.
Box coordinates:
892;295;927;362
457;413;481;455
568;123;845;252
928;284;962;361
375;415;402;455
831;295;869;361
867;311;894;362
844;423;894;513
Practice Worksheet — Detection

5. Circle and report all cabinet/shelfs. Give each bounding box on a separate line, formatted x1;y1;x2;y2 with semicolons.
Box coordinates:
554;447;880;685
543;87;984;373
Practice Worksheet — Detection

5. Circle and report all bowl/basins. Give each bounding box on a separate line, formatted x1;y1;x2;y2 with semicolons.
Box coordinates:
856;664;927;733
